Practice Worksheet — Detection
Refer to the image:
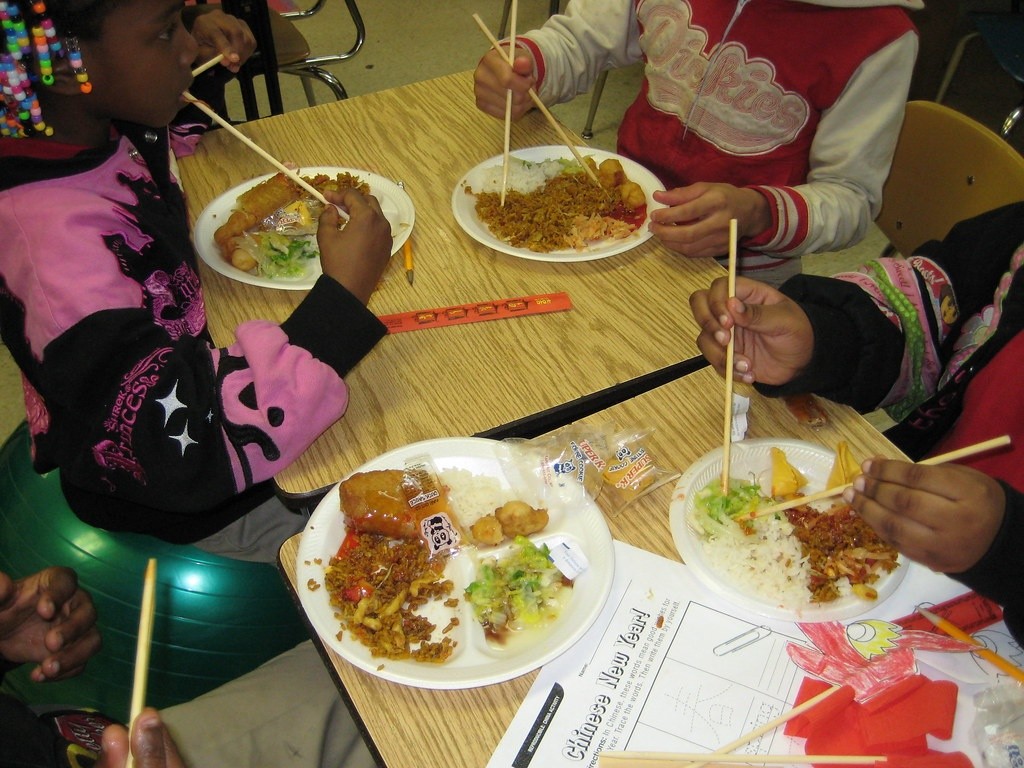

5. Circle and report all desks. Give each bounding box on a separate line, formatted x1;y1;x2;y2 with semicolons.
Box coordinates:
179;69;729;516
278;364;915;768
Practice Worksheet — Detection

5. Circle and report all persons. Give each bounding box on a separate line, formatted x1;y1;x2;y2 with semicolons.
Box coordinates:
0;0;393;569
474;0;926;258
689;201;1023;647
0;564;386;768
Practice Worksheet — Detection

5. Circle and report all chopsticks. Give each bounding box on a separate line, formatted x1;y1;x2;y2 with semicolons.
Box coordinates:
595;677;885;768
722;215;1009;524
473;0;611;207
182;52;349;221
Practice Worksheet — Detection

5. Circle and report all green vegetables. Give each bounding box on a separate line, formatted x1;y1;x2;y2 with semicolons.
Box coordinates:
236;231;319;279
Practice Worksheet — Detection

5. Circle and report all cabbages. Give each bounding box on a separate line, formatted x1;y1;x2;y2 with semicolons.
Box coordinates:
697;483;776;525
466;537;557;624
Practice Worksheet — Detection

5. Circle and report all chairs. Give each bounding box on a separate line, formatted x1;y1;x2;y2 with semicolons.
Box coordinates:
181;0;365;129
874;100;1024;257
934;12;1024;138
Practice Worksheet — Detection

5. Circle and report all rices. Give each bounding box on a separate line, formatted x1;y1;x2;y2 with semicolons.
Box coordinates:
483;159;562;194
703;527;811;602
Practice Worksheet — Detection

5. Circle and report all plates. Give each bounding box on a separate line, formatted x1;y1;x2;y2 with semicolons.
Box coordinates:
296;437;614;691
192;165;415;291
669;437;909;624
452;144;670;263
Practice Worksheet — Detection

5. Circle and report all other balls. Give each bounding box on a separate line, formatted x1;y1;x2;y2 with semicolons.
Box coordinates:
0;419;318;730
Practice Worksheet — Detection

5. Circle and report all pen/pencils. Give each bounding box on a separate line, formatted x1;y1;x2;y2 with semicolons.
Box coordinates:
913;605;1024;681
395;181;416;287
